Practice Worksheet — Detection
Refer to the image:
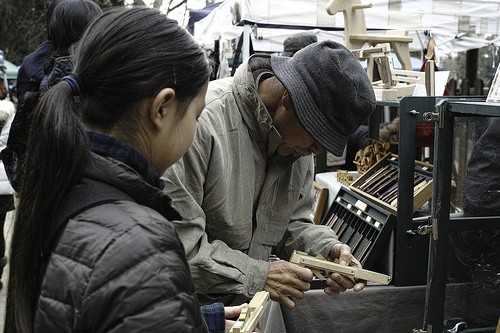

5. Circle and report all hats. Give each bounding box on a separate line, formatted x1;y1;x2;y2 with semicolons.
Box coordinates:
282;32;317;56
270;39;377;157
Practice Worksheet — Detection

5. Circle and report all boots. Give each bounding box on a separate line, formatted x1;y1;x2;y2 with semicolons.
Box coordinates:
0;256;7;290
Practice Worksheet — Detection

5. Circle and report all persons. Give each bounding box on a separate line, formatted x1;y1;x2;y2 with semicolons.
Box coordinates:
0;0;500;333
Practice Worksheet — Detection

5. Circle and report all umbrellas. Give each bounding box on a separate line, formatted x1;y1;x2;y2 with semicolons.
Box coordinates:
0;59;19;80
